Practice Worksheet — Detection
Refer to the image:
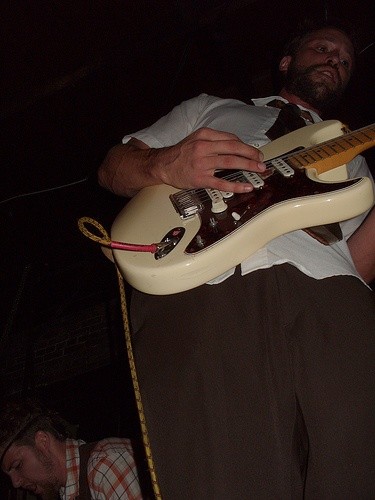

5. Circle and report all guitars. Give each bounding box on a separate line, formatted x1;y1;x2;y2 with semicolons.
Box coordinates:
110;120;375;296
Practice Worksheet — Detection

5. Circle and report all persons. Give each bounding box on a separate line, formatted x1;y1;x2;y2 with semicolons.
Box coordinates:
97;24;375;500
0;398;143;500
